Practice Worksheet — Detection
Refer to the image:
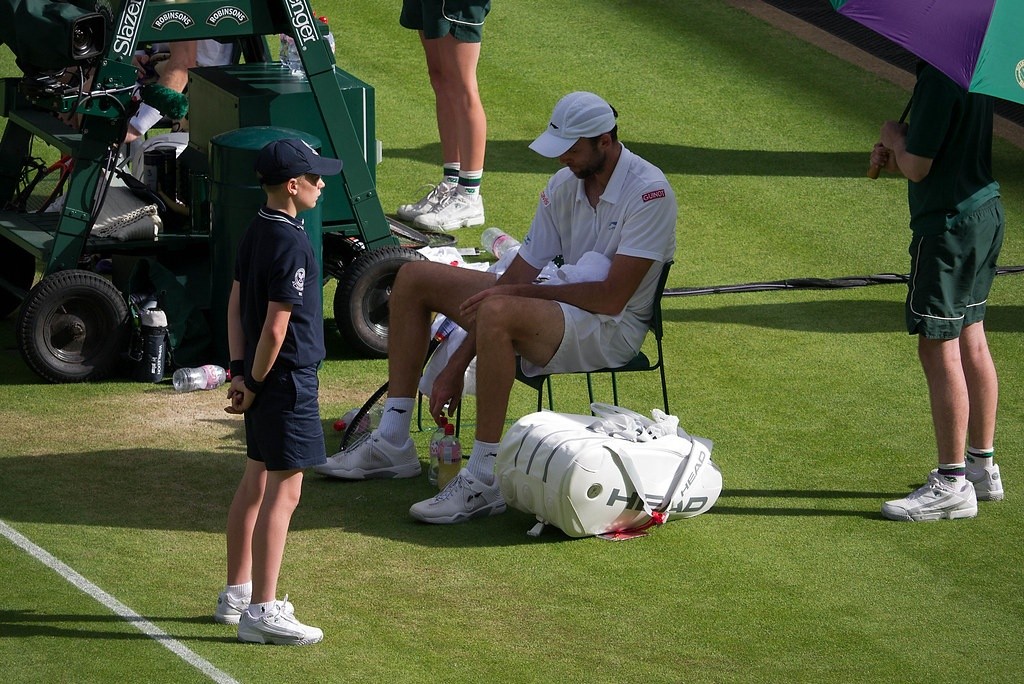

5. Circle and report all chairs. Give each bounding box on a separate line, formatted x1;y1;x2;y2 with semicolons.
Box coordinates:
418;253;675;460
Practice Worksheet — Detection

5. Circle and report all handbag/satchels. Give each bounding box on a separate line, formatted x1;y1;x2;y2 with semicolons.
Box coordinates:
126;305;173;384
587;402;679;442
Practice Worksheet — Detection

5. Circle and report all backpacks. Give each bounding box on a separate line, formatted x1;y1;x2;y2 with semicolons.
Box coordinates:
496;410;722;537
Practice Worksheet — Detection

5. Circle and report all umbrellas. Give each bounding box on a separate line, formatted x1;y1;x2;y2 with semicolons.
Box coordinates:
829;0;1024;179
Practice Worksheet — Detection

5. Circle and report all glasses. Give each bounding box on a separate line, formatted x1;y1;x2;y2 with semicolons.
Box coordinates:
302;171;320;186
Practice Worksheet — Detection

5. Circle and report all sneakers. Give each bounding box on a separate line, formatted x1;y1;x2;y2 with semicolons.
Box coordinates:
928;463;1004;501
397;181;456;221
238;594;323;645
215;593;250;625
409;468;506;523
414;186;485;231
313;429;422;478
882;477;977;520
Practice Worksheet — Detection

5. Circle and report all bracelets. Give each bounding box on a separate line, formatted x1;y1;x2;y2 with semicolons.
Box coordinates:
229;360;245;379
244;371;264;394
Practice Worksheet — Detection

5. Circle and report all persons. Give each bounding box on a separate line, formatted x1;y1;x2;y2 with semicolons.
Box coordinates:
30;39;233;214
870;58;1005;522
312;91;677;524
397;0;492;234
214;138;343;646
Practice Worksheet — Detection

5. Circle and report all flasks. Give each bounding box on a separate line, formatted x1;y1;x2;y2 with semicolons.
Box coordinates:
130;299;169;383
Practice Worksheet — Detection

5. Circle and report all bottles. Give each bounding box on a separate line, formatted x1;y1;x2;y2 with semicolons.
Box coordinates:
480;227;520;260
317;17;336;74
429;417;451;486
334;403;387;433
436;423;462;491
279;33;308;79
172;365;233;392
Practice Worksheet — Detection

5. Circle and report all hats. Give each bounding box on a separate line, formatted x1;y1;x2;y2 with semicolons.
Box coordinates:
528;91;617;158
255;139;343;184
